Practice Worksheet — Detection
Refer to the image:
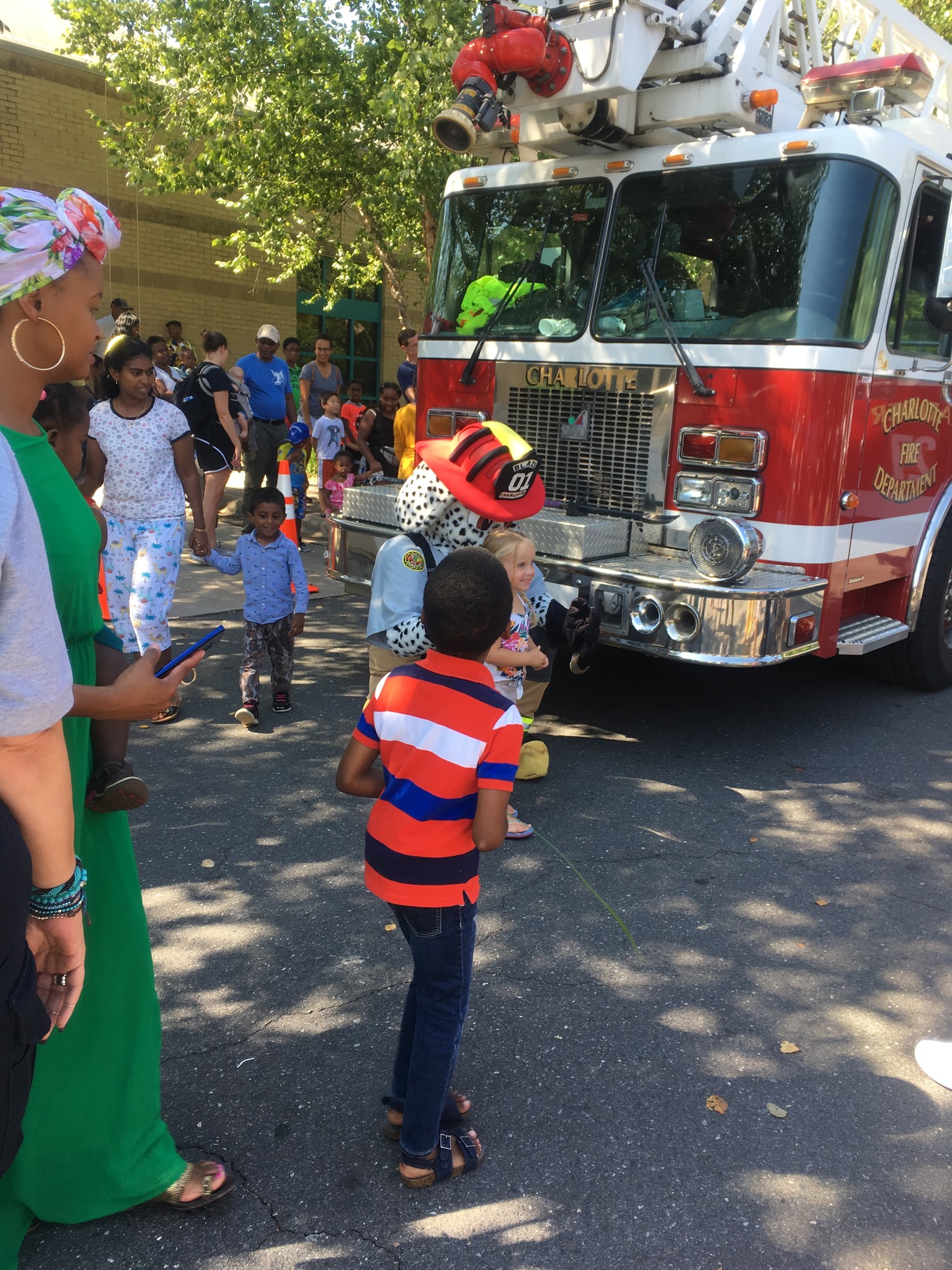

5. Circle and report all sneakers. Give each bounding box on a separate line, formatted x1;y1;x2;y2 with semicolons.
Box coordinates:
235;700;261;726
272;692;292;713
297;535;310;552
213;543;235;556
188;551;214;566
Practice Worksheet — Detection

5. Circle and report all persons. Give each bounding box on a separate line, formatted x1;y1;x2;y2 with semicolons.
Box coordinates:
192;486;309;726
80;333;209;722
43;383;150;817
0;181;237;1269
86;297;417;560
0;427;86;1198
334;548;523;1186
479;527;549;837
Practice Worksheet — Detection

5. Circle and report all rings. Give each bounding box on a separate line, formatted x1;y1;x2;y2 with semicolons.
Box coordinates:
47;1011;59;1014
53;974;67;986
201;551;204;552
63;1004;76;1007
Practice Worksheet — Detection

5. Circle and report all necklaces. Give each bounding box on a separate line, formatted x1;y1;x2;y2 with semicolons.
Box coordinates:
318;363;329;376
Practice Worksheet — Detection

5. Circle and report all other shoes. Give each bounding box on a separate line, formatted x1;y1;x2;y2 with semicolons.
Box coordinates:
323;548;329;560
306;496;312;502
85;761;148;814
320;513;327;518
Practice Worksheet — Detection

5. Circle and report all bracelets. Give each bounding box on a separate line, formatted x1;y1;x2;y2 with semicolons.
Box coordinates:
25;854;92;927
194;529;207;532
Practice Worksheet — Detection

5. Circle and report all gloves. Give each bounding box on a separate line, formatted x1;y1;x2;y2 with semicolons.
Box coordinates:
564;595;604;675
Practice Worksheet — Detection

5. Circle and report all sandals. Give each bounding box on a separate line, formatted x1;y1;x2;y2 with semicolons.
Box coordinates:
399;1128;485;1189
153;1157;236;1211
383;1087;473;1138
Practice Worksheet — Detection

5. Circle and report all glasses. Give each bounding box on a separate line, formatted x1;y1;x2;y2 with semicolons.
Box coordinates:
258;340;275;345
226;347;231;355
314;347;331;353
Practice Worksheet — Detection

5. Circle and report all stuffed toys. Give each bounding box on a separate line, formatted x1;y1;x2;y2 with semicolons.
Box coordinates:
366;419;548;779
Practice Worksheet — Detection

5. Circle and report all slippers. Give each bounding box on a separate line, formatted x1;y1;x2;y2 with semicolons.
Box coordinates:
150;695;183;723
505;805;534;840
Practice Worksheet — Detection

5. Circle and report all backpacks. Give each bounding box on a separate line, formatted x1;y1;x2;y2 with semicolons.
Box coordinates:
171;364;215;436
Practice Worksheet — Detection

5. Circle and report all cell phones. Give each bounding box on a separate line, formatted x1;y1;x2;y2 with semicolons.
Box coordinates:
155;625;225;679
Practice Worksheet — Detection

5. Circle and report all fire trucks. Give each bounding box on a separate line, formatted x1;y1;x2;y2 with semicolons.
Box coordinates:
321;0;952;694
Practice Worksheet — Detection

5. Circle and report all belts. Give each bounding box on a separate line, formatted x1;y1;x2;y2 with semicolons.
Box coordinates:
251;416;285;426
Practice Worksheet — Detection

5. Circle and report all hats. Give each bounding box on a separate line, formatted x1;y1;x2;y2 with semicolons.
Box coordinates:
257;324;280;343
414;419;547;523
285;422;309;443
110;298;134;310
166;320;182;327
